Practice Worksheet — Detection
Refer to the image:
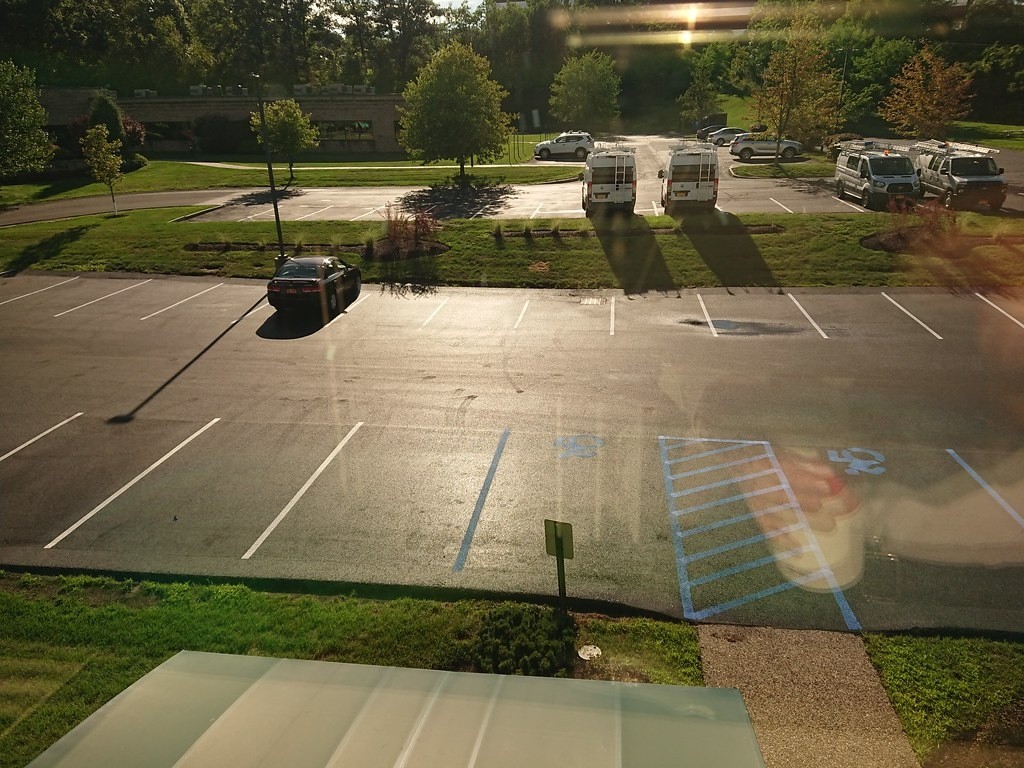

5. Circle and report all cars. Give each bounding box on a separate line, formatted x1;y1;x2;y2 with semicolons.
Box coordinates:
268;254;361;311
696;125;728;140
707;127;749;145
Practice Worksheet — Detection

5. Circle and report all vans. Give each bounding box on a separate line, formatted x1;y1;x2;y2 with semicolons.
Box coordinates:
914;141;1006;210
835;137;921;208
658;141;719;210
582;142;636;218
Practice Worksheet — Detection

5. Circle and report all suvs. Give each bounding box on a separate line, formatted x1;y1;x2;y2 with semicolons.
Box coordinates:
729;132;802;160
534;130;593;159
